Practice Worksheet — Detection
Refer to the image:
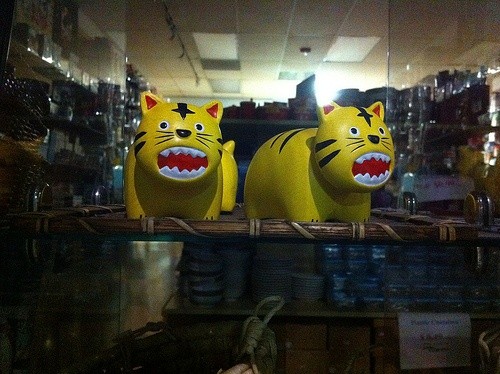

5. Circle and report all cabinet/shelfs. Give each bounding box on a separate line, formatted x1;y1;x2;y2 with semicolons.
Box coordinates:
45;78;108;179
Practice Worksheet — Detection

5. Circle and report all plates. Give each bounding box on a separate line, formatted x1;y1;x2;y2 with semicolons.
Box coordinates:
250;255;325;305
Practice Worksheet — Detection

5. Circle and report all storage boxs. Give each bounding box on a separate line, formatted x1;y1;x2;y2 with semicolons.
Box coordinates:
270;318;500;374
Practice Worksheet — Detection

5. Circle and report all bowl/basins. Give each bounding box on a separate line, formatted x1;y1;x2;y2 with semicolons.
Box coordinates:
324;245;500;314
186;251;226;305
366;86;433;122
